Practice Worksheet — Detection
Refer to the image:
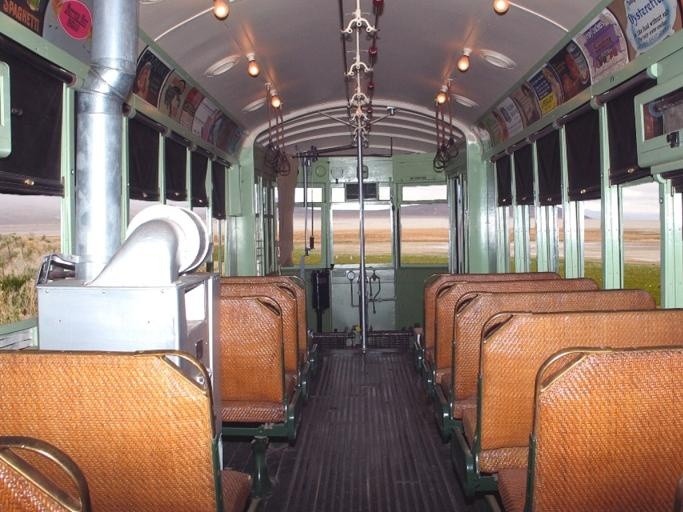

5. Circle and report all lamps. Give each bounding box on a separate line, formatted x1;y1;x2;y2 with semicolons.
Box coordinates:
491;1;511;16
268;88;281;110
244;52;261;78
457;46;473;74
212;1;230;21
433;82;450;105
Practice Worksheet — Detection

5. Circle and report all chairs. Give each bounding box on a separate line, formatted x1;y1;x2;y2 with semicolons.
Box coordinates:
0;347;259;512
213;269;317;501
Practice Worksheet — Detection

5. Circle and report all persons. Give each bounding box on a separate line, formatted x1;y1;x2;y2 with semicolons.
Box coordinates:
490;110;510;142
163;80;187;107
543;68;564;109
135;61;154;98
520;85;539;127
566;56;583;92
